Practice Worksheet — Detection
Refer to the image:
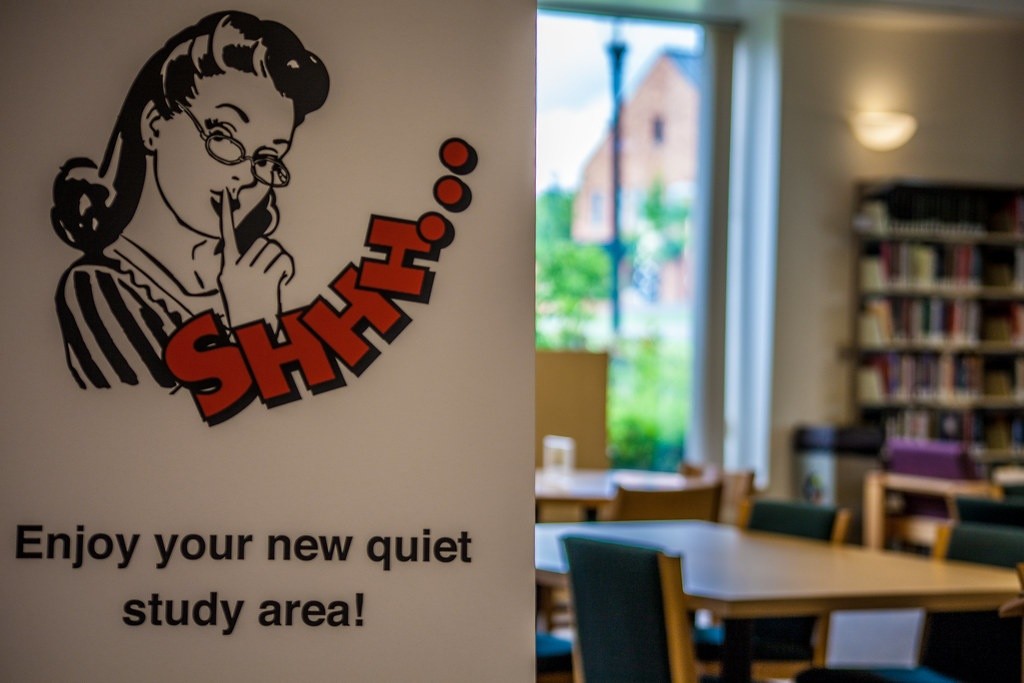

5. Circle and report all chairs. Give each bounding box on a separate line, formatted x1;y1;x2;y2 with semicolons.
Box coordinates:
560;533;695;683
859;431;1004;552
692;498;853;683
781;520;1024;683
610;481;722;528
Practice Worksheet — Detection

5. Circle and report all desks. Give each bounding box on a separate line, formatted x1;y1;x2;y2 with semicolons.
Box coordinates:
536;465;721;522
535;521;1024;683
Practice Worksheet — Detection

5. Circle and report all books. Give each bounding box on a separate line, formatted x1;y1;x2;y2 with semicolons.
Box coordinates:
858;237;1024;459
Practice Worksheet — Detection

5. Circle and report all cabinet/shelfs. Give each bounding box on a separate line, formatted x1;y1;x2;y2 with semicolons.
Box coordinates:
850;176;1024;463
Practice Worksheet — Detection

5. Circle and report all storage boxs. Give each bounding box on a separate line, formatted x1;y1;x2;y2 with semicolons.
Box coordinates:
787;426;872;507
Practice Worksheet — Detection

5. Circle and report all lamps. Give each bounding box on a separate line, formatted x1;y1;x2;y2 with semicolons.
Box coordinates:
851;113;918;151
